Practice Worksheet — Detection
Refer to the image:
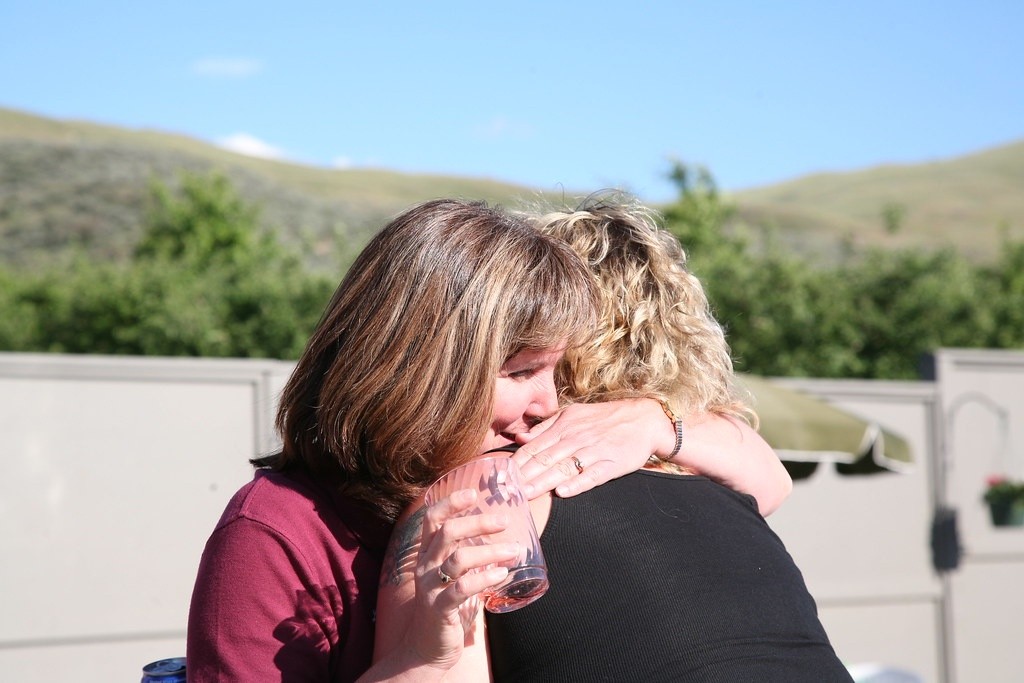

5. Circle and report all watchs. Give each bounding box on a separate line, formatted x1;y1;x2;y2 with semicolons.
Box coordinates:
660;400;683;462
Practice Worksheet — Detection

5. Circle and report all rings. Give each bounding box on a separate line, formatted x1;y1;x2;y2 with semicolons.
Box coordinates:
571;456;585;474
438;566;456;583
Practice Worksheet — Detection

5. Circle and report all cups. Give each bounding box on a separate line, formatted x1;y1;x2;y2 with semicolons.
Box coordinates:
425;453;547;615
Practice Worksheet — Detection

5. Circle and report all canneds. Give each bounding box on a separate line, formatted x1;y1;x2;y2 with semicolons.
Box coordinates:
141;657;188;683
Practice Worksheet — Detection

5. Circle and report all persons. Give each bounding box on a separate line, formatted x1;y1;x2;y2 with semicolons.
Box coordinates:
373;188;857;683
186;199;793;682
